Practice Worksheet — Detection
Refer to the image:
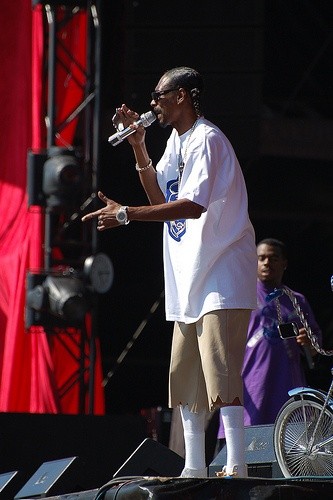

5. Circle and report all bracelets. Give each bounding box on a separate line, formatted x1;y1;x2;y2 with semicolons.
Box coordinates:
135;158;152;172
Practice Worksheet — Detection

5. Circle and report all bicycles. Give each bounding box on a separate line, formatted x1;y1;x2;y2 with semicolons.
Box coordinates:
272;368;333;478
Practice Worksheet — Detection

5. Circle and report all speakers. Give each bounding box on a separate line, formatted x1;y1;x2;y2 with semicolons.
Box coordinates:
0;470;21;500
114;436;185;477
14;456;97;498
210;420;317;480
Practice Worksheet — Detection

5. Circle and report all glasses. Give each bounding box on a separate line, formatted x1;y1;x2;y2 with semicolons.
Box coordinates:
151;88;190;102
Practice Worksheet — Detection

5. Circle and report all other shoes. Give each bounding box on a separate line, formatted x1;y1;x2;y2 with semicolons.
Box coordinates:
179;469;208;478
216;463;249;478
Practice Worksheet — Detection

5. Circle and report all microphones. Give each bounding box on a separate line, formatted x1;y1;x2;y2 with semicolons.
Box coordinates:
108;109;157;146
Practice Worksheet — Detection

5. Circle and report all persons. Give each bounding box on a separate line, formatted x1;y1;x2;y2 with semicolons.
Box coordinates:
212;238;322;461
81;65;259;478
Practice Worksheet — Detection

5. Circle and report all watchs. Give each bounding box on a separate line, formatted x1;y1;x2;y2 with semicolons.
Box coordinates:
116;206;130;225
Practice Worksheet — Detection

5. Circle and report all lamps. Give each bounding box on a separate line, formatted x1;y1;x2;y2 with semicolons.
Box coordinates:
24;273;90;328
27;148;93;214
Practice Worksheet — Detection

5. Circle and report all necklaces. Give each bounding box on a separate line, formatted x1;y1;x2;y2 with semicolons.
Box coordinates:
178;121;196;173
